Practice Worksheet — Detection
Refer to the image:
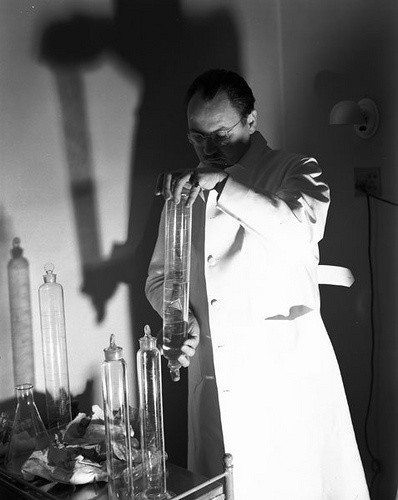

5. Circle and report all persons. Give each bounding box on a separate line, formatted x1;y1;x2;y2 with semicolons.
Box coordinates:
144;69;371;500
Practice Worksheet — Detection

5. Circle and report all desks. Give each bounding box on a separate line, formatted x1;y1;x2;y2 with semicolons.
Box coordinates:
0;397;235;499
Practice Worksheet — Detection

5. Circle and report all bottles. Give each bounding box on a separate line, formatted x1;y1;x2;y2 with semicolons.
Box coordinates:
163;191;192;377
102;334;134;500
137;324;167;499
6;384;51;476
38;262;72;429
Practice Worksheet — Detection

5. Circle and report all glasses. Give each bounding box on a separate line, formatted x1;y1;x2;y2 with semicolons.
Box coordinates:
186;114;246;145
